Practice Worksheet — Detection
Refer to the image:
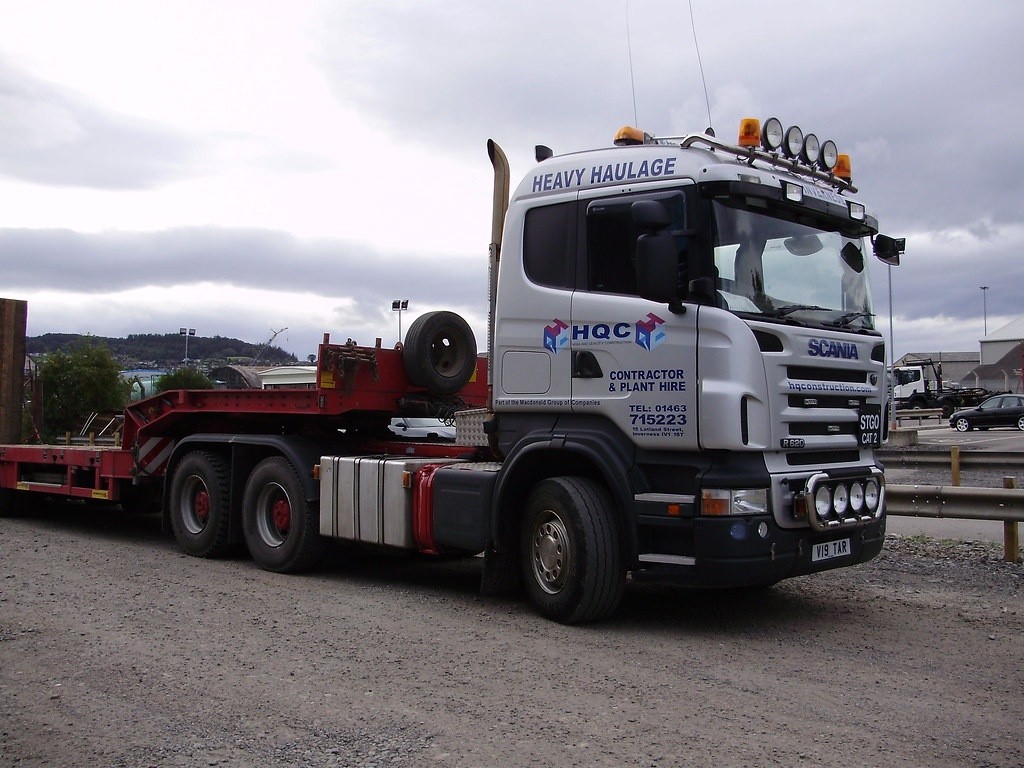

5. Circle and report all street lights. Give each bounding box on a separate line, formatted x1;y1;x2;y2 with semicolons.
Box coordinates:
179;328;196;370
869;238;906;433
391;298;409;343
979;285;990;337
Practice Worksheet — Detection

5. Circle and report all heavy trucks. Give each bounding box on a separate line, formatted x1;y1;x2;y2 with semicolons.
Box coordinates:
0;114;907;625
886;351;1013;419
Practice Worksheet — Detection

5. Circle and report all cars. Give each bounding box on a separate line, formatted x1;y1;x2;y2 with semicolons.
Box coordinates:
949;394;1024;432
